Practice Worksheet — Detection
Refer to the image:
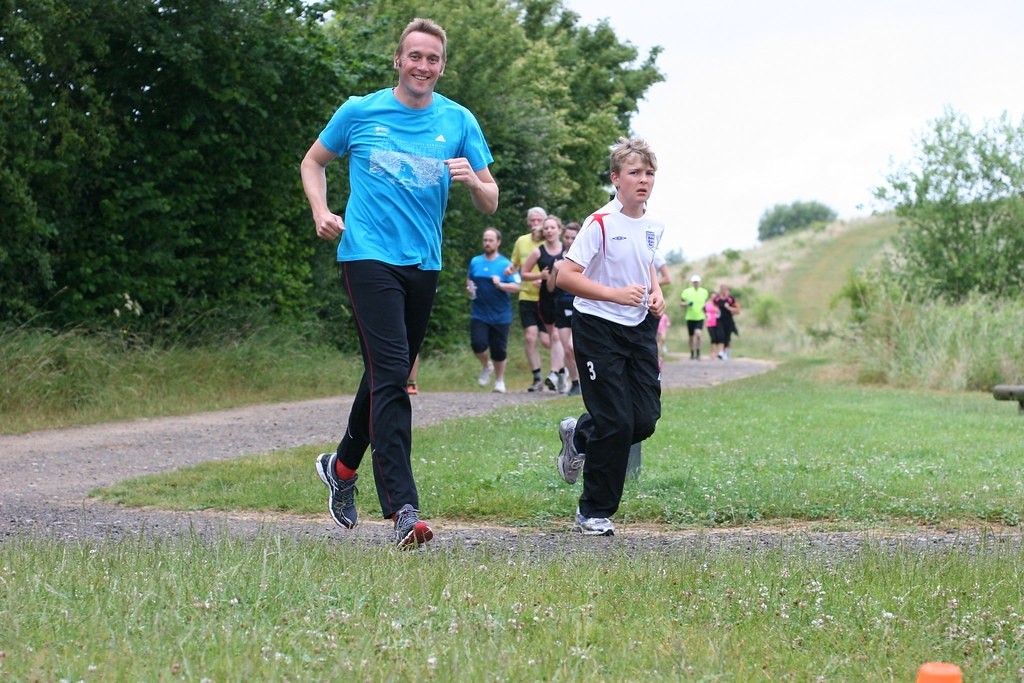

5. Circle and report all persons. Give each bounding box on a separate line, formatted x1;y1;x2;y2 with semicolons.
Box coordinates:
680;275;740;361
301;18;499;556
557;141;664;536
504;206;671;397
465;227;521;393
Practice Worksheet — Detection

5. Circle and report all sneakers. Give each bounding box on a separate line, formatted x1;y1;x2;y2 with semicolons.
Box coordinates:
478;361;494;387
572;506;615;536
316;453;360;529
492;381;505;393
556;417;585;485
393;504;434;549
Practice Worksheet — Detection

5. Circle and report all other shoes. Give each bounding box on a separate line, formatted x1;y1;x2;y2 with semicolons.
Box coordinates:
407;382;418;395
718;352;728;361
559;367;569;393
544;372;558;391
569;382;580;395
528;377;544;391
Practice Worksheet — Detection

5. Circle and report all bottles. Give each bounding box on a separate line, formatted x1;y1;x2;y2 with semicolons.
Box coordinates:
469;281;476;300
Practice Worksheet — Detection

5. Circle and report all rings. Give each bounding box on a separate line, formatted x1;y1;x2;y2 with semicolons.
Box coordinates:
454;169;458;176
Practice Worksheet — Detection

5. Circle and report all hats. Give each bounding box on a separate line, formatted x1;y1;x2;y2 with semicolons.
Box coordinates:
691;275;701;282
